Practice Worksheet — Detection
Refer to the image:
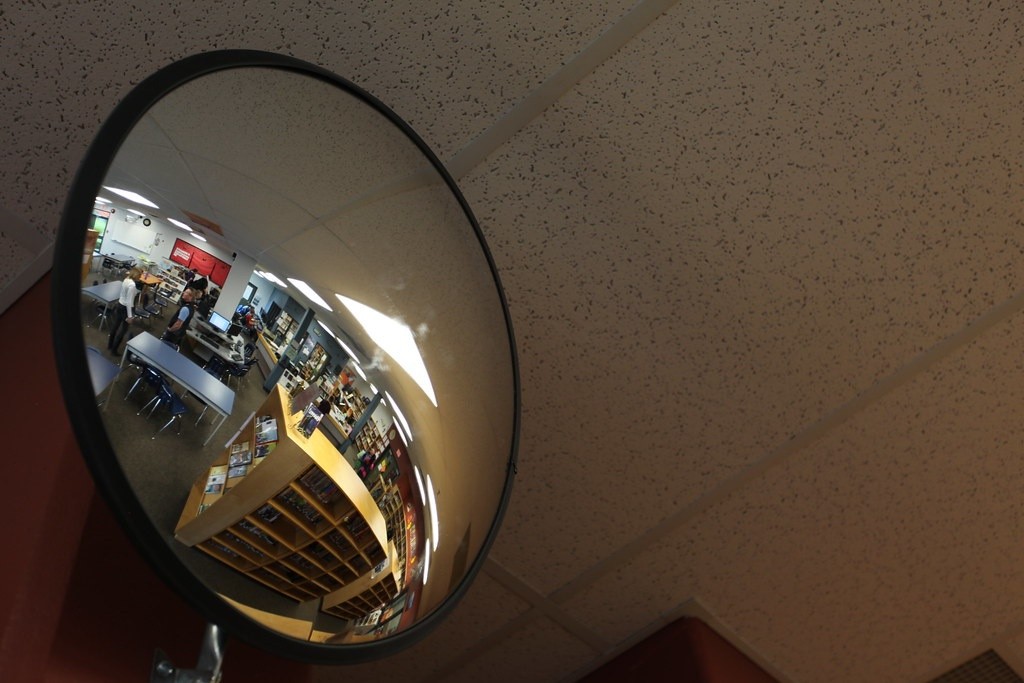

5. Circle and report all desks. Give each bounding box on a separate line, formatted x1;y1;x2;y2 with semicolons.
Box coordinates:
137;272;162;293
301;385;348;445
103;331;235;446
81;281;142;331
186;311;244;365
102;255;135;278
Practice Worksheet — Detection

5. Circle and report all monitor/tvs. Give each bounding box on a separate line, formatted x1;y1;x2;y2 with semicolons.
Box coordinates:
197;294;243;340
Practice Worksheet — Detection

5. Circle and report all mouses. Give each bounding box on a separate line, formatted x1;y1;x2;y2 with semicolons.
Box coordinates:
196;334;198;337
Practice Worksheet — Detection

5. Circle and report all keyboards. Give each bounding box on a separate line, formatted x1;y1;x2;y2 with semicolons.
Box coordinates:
232;354;243;361
237;341;243;347
200;334;219;349
235;344;241;354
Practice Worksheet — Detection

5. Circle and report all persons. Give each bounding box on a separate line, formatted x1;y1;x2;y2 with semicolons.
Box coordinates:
107;265;142;358
357;448;377;481
158;268;221;349
236;303;259;340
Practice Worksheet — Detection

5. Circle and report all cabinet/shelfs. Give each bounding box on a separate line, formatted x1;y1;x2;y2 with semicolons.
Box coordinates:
321;541;401;621
366;435;386;460
386;479;408;586
355;610;382;628
174;383;388;602
381;485;404;520
343;389;386;455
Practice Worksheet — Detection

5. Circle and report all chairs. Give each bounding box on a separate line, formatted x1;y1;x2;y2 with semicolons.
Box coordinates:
124;364;171;421
244;342;256;355
103;252;137;276
244;356;260;376
181;366;222;426
202;354;224;380
121;333;146;378
151;289;168;316
224;366;250;391
143;294;160;320
132;293;152;329
137;385;186;439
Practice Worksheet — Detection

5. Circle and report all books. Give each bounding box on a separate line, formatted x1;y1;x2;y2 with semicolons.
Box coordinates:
195;403;371;578
